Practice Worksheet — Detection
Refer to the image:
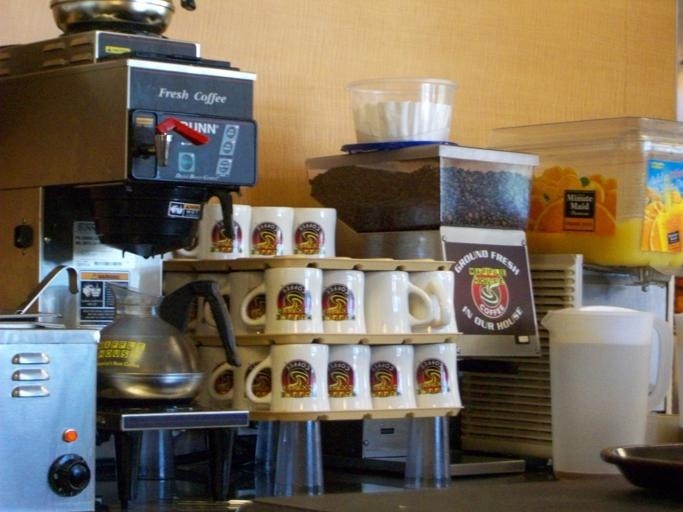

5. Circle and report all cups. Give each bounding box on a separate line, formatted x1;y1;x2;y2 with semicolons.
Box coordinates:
163;267;451;337
176;204;339;258
195;335;467;415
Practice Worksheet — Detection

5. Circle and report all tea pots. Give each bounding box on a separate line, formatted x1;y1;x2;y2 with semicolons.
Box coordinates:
96;280;241;404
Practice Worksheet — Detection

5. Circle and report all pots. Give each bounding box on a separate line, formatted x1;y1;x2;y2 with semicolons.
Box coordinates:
48;0;195;33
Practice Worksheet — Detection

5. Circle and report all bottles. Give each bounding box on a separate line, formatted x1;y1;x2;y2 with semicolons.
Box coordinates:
542;307;673;478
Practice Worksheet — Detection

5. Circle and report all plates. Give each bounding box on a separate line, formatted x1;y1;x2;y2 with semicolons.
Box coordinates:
599;442;683;491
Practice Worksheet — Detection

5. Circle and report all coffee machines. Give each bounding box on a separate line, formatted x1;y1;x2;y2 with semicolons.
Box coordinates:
325;226;543;481
0;35;259;331
